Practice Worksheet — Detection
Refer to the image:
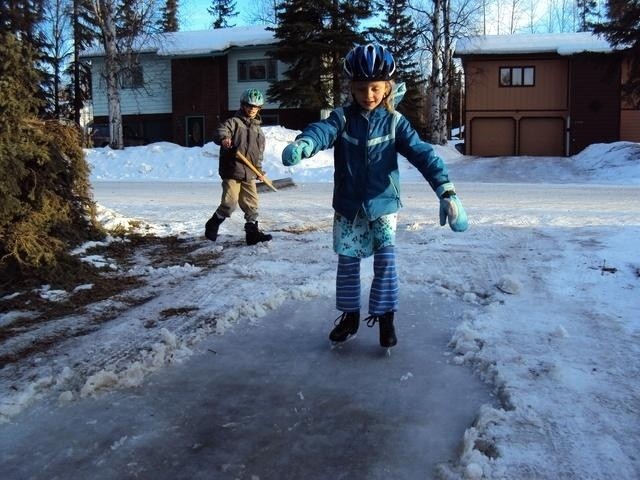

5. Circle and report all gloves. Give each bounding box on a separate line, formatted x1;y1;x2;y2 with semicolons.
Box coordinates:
434;180;470;234
279;135;314;166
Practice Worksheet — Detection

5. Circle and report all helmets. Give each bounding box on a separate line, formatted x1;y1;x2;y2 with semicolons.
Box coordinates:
238;87;265;108
342;43;396;82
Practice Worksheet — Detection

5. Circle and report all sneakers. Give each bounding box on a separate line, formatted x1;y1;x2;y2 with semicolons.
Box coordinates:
372;312;398;347
327;310;359;342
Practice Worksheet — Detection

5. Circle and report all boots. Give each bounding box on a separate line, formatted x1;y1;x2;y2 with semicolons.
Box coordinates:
244;219;273;246
202;210;225;242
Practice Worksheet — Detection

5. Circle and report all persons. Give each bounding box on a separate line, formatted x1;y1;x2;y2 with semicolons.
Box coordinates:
280;42;469;349
204;87;273;245
